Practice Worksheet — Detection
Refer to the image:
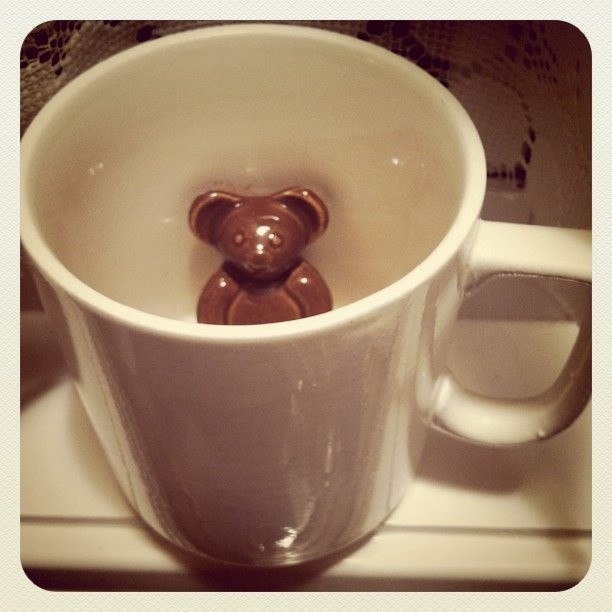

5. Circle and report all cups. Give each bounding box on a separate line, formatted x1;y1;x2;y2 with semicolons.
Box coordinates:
21;23;591;569
